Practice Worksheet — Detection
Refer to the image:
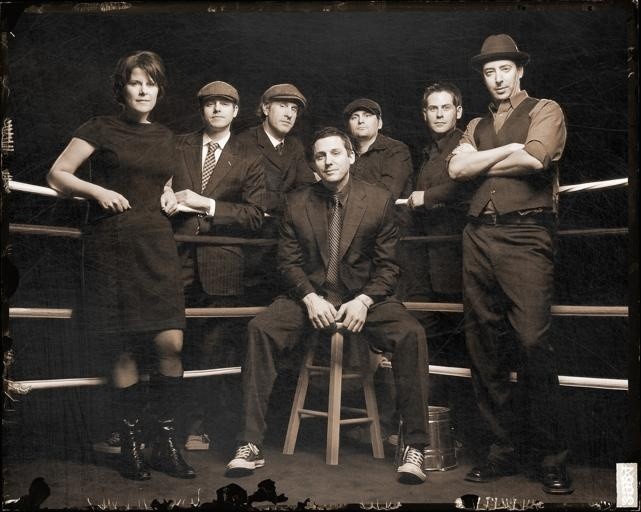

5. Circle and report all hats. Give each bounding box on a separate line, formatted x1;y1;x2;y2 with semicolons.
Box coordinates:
197;81;240;105
262;83;307;112
343;99;381;122
470;33;531;72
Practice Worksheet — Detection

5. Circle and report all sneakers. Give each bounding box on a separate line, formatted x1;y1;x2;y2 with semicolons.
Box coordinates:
396;445;427;484
225;442;265;477
92;430;122;454
185;433;211;450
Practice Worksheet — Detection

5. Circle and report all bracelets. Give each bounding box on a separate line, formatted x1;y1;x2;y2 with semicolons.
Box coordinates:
354;296;370;310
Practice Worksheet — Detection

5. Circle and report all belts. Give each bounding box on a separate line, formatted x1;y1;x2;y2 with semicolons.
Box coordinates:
468;214;540;225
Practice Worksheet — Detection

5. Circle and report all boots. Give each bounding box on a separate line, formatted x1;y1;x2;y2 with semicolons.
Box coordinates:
117;415;151;481
151;416;196;479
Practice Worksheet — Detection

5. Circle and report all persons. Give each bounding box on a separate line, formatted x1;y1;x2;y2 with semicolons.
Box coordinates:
83;80;273;456
45;47;195;483
235;79;322;422
405;78;491;460
344;96;449;443
223;126;432;484
445;33;574;495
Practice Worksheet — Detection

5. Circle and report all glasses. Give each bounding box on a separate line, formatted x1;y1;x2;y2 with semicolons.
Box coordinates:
425;104;451;112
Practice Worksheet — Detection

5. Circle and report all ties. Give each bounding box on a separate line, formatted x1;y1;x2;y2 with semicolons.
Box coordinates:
275;142;283;153
325;193;342;308
194;141;219;236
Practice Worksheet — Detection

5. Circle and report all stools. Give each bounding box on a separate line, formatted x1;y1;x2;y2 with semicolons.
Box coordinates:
282;318;388;466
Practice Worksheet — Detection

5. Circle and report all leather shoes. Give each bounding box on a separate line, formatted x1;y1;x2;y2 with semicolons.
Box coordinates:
464;461;512;482
535;467;569;493
